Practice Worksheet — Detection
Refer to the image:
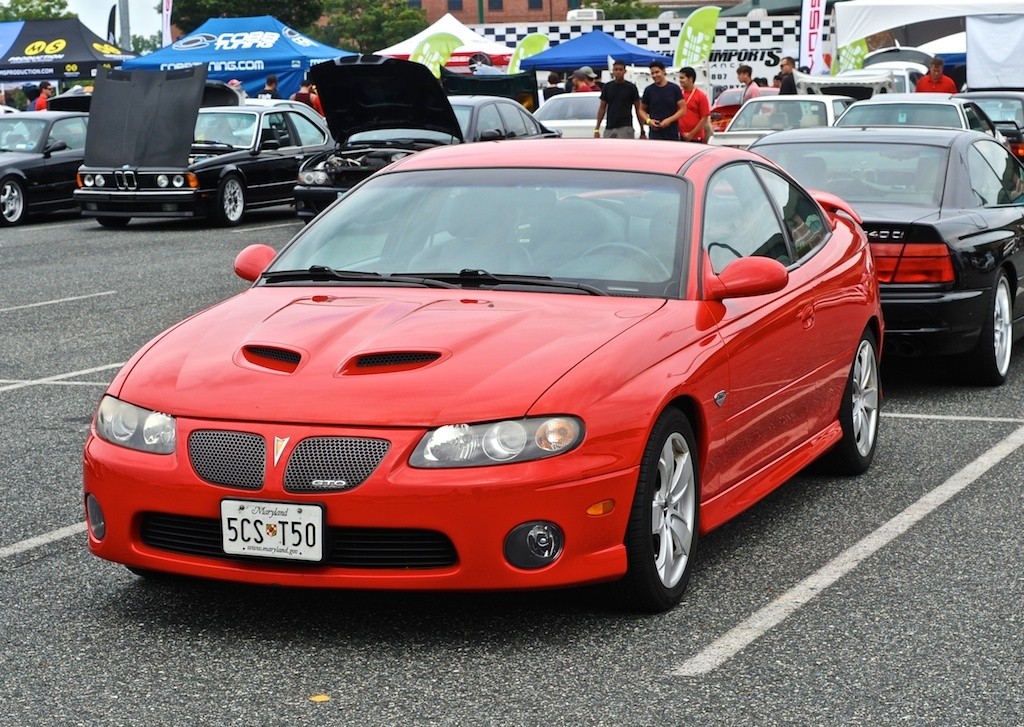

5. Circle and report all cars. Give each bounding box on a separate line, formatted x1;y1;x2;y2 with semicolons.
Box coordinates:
706;95;856;198
948;92;1024;167
709;124;1024;386
294;55;564;237
82;138;884;612
0;111;90;228
532;92;650;139
73;61;341;228
831;67;927;123
808;99;1015;205
710;87;780;133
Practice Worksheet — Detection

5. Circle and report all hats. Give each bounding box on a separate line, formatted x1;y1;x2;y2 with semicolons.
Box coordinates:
568;70;585;79
228;79;242;87
580;66;598;78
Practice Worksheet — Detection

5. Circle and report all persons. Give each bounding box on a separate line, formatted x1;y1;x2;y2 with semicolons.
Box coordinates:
679;67;711;144
778;56;798;95
290;80;325;122
257;75;281;99
29;98;36;111
594;59;647;139
0;90;4;105
773;76;781;87
915;59;957;93
543;66;601;102
36;81;52;111
737;66;760;105
638;61;686;141
229;78;249;98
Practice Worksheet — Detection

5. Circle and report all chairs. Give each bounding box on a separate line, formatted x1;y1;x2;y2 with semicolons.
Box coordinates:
800;115;819;128
408;185;679;284
262;129;280;141
909;109;944;126
915;158;940;190
750;113;787;128
804;157;827;180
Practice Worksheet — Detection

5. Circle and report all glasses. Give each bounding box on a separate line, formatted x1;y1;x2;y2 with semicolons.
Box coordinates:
781;64;788;68
45;88;51;90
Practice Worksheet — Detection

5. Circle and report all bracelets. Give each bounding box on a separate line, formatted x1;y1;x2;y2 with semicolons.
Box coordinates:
646;118;651;124
594;129;599;132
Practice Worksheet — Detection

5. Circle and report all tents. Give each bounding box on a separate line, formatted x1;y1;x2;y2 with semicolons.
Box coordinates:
0;15;674;100
